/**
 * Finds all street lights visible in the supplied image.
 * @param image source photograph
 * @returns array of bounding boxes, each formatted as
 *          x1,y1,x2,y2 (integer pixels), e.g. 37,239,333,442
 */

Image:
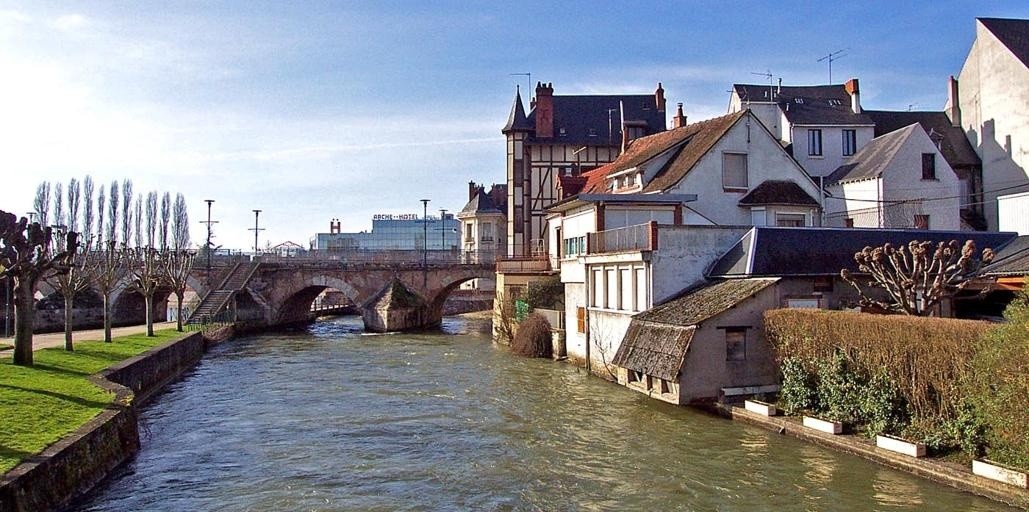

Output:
420,199,431,288
51,224,61,253
204,199,215,286
252,209,262,263
26,212,37,225
4,266,13,337
438,208,449,264
